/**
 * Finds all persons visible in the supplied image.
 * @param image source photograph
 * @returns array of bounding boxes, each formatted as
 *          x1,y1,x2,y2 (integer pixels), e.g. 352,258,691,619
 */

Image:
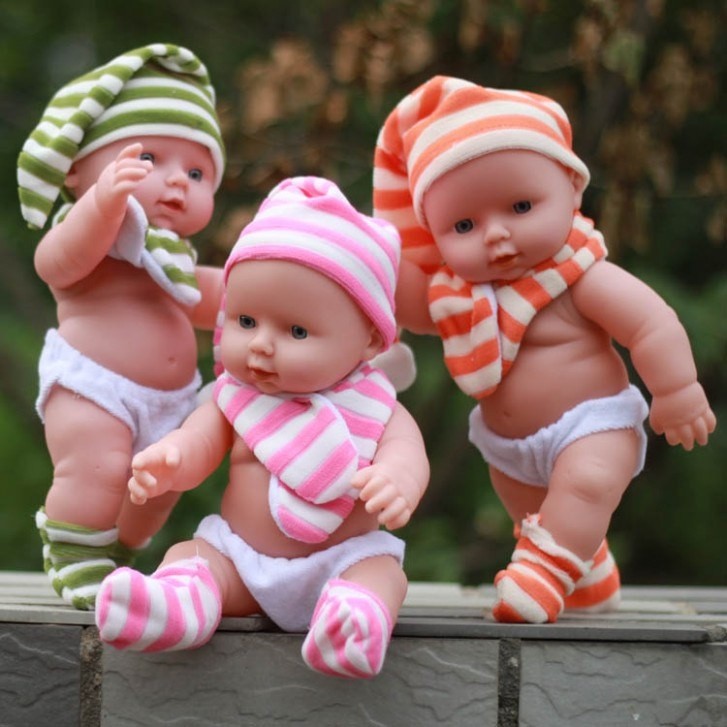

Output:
18,43,232,613
95,176,429,676
372,74,717,621
